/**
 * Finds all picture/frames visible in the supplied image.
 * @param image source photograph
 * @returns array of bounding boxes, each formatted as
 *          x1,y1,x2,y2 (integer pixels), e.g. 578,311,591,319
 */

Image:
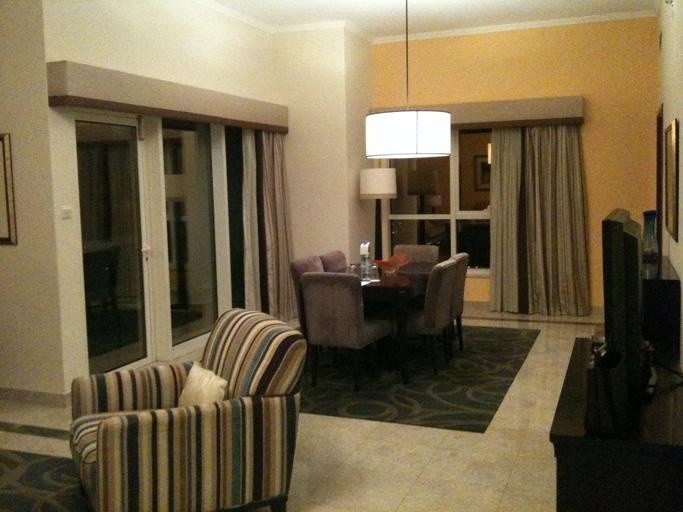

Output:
472,153,492,193
664,117,680,244
0,131,19,248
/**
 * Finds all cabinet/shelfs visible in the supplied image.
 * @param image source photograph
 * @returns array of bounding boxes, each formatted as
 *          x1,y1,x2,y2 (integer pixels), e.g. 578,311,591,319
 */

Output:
549,336,683,511
640,253,681,363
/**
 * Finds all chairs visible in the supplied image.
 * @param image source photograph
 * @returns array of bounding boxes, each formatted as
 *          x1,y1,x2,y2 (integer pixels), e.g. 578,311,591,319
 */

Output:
449,251,470,358
289,255,327,332
392,243,440,271
298,270,391,393
319,249,350,273
405,258,459,377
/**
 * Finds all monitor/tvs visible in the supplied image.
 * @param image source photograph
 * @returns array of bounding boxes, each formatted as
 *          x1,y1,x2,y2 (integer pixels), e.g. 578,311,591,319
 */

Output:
600,206,645,440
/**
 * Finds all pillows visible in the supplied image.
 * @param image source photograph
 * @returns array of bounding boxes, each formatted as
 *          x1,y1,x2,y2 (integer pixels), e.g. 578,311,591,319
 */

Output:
176,357,229,409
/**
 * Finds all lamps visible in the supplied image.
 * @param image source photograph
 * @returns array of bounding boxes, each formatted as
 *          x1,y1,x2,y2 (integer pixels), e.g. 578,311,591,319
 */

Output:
363,0,453,160
359,166,399,261
405,167,437,245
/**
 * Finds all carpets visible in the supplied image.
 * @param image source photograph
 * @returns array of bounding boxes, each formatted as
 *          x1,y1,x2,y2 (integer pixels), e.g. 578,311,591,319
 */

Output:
0,446,92,511
296,323,541,435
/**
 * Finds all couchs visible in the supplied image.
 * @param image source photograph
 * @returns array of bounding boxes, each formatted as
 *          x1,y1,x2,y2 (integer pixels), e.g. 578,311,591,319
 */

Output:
68,306,311,512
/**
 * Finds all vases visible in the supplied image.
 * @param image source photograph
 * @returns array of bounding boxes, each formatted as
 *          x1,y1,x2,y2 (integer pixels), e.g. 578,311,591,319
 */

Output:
640,209,661,266
